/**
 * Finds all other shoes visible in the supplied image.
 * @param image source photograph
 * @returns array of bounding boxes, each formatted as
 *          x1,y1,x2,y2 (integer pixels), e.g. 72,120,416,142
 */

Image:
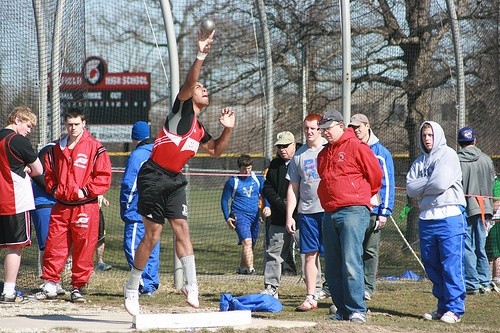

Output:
348,312,366,324
236,267,255,275
0,293,24,304
423,311,442,320
56,284,66,295
440,311,461,323
35,290,57,300
96,262,112,271
490,282,500,293
480,288,490,295
181,286,199,309
466,289,479,295
122,285,139,316
325,313,345,322
258,287,279,300
296,294,317,312
317,291,332,300
365,291,371,301
69,290,86,303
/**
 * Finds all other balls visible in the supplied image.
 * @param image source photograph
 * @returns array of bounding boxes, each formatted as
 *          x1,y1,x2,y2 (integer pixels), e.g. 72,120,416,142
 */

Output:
201,19,215,33
423,313,432,321
329,305,338,316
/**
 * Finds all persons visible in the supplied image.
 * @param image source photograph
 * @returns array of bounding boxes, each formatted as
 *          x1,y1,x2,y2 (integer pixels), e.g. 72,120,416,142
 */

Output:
457,126,500,294
31,108,113,303
260,130,321,301
122,30,235,316
348,114,395,300
119,119,160,296
317,109,384,323
285,113,329,311
221,154,260,275
406,121,467,323
0,105,43,305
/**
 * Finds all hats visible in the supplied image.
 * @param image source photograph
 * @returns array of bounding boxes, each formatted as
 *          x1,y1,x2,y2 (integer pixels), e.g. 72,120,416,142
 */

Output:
347,114,369,128
316,110,343,131
274,131,294,145
131,121,150,141
458,126,476,141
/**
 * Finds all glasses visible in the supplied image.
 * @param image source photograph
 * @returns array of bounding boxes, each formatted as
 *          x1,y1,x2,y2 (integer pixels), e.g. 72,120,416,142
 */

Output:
276,142,293,149
320,123,339,133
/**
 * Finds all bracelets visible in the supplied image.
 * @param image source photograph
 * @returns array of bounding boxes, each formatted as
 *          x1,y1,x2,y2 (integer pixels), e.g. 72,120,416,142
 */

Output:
196,51,209,61
